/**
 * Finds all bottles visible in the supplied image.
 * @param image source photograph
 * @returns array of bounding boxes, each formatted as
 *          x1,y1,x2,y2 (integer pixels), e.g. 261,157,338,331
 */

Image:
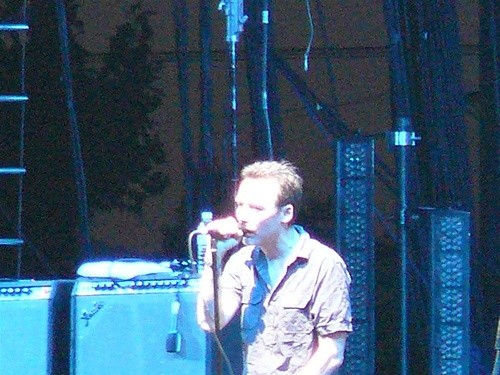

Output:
197,212,212,273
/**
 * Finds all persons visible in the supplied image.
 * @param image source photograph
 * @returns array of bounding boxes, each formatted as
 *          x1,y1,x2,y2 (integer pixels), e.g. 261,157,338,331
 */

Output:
198,160,353,375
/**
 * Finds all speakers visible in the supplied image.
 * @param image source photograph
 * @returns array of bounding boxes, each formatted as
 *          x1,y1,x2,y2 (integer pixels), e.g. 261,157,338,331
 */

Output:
0,274,212,375
